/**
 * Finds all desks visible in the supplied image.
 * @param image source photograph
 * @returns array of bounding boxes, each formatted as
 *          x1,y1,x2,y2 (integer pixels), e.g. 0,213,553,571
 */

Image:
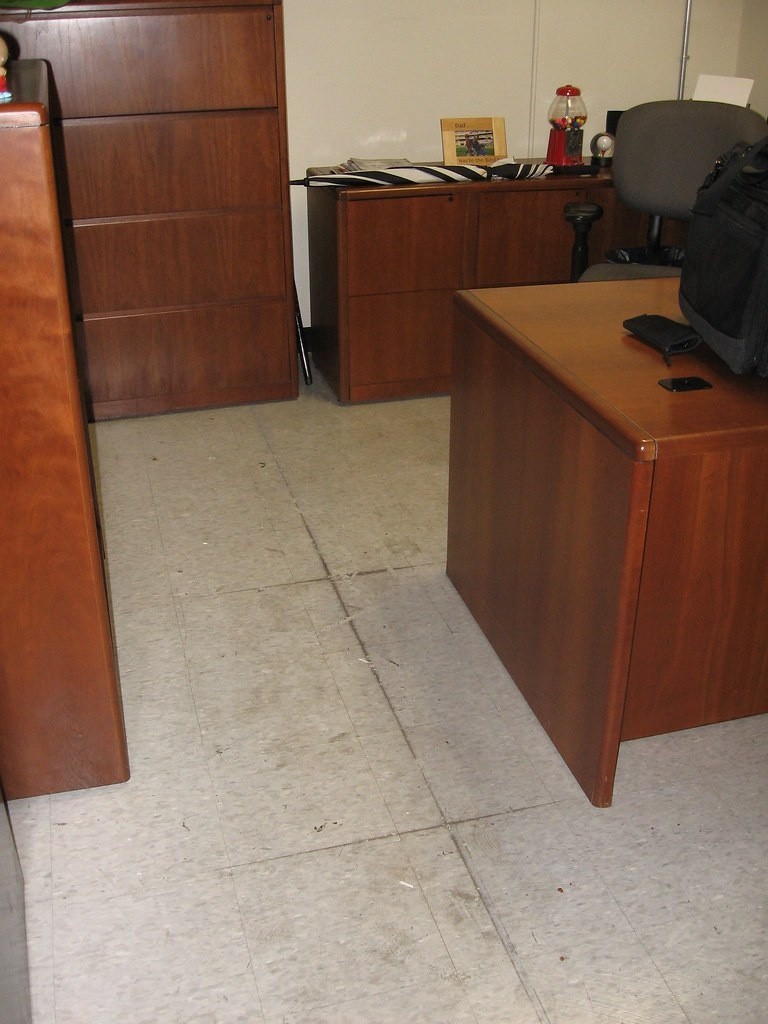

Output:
446,278,767,807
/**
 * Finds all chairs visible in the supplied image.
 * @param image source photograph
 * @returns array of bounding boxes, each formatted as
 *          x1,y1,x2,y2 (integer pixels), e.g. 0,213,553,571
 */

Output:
560,100,767,282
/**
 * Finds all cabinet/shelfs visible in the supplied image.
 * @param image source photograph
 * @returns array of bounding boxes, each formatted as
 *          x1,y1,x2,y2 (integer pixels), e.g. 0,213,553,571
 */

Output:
306,158,654,408
0,0,301,424
0,56,129,807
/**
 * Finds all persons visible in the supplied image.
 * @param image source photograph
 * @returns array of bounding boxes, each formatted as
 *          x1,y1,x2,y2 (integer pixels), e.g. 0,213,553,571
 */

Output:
464,134,484,156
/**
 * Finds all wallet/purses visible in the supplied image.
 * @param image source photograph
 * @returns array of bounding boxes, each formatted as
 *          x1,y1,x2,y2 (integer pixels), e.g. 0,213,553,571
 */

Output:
624,313,702,367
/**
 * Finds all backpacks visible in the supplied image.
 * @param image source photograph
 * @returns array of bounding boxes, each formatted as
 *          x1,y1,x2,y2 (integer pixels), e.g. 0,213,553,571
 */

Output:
678,136,768,378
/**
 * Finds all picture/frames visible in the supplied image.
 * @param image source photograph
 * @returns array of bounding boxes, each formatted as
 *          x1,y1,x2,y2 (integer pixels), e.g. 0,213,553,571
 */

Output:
440,117,508,166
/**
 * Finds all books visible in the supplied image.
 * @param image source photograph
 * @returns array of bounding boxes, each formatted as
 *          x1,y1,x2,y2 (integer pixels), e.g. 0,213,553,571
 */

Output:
331,158,413,174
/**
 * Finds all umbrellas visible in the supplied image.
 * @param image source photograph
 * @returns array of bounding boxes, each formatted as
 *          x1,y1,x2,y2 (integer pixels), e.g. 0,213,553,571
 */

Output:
287,163,599,188
